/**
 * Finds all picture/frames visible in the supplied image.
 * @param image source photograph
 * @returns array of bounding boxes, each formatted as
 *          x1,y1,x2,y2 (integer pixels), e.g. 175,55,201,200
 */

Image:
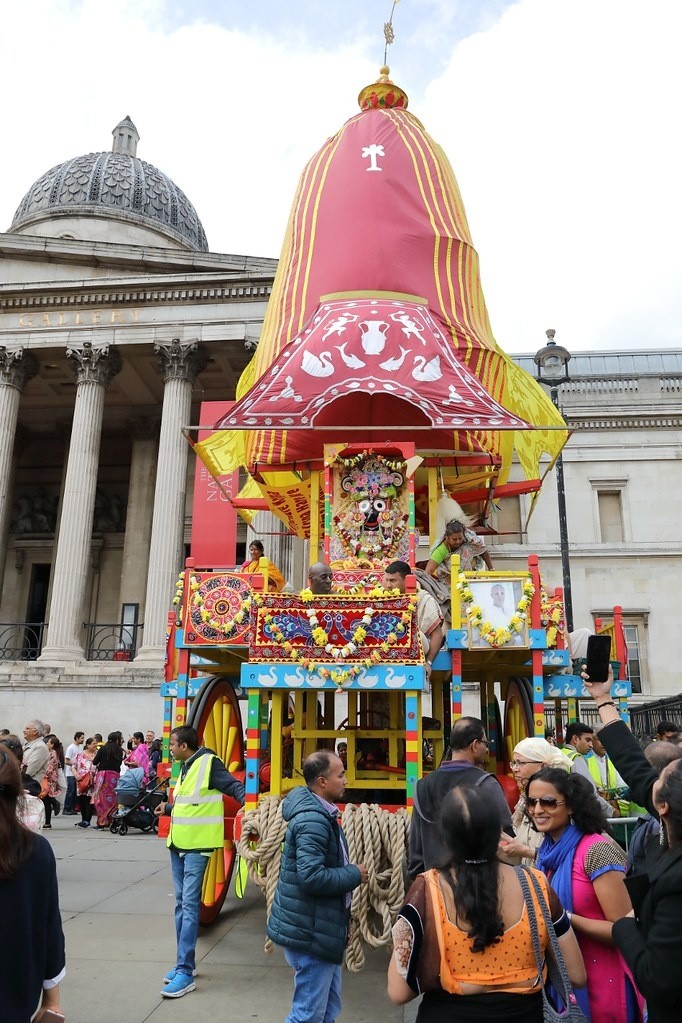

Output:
463,577,532,651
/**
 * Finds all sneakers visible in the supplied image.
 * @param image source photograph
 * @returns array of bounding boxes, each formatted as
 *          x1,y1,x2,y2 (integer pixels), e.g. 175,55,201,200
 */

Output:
160,966,197,997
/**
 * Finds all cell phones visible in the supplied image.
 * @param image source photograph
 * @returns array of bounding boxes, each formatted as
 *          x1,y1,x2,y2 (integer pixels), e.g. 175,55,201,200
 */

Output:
585,634,611,683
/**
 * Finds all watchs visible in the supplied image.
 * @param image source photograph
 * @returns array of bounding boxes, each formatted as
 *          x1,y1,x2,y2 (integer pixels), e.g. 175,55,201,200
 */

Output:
426,658,433,666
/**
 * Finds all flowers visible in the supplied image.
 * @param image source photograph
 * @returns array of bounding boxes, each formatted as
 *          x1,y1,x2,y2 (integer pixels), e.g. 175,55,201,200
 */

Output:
456,569,536,648
539,574,562,648
253,574,419,692
172,571,253,638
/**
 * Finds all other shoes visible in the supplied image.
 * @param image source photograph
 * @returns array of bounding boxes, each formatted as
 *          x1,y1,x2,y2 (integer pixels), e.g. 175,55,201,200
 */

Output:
53,801,60,816
93,825,110,830
39,824,52,829
74,821,91,828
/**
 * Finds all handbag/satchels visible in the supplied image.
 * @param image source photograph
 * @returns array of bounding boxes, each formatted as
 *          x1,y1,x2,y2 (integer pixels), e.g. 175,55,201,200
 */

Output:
514,864,586,1023
38,778,49,798
77,771,94,793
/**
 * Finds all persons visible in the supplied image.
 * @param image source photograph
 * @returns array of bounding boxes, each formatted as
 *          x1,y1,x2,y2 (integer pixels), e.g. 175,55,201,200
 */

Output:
337,742,387,771
425,519,496,629
0,743,66,1023
0,720,163,830
385,561,444,679
386,659,682,1023
154,726,245,998
267,751,369,1023
240,540,285,594
266,689,325,778
484,584,514,631
306,562,340,594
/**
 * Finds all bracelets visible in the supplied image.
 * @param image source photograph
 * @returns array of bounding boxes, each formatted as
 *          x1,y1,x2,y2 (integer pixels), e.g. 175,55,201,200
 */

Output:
291,723,295,729
597,702,616,709
489,568,495,571
566,910,572,924
529,846,535,863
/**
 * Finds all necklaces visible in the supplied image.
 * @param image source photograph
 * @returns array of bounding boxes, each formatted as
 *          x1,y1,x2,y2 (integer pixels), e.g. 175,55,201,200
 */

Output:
249,561,259,583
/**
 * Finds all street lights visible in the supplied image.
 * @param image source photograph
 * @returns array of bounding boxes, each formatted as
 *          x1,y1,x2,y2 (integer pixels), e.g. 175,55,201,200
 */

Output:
533,329,579,633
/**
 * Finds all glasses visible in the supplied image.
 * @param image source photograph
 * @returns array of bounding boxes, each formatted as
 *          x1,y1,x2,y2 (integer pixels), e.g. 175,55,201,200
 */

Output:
478,740,491,747
509,760,543,770
524,797,565,812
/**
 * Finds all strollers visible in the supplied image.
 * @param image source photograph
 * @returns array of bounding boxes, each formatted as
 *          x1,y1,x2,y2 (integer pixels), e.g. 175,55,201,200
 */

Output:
108,766,171,835
